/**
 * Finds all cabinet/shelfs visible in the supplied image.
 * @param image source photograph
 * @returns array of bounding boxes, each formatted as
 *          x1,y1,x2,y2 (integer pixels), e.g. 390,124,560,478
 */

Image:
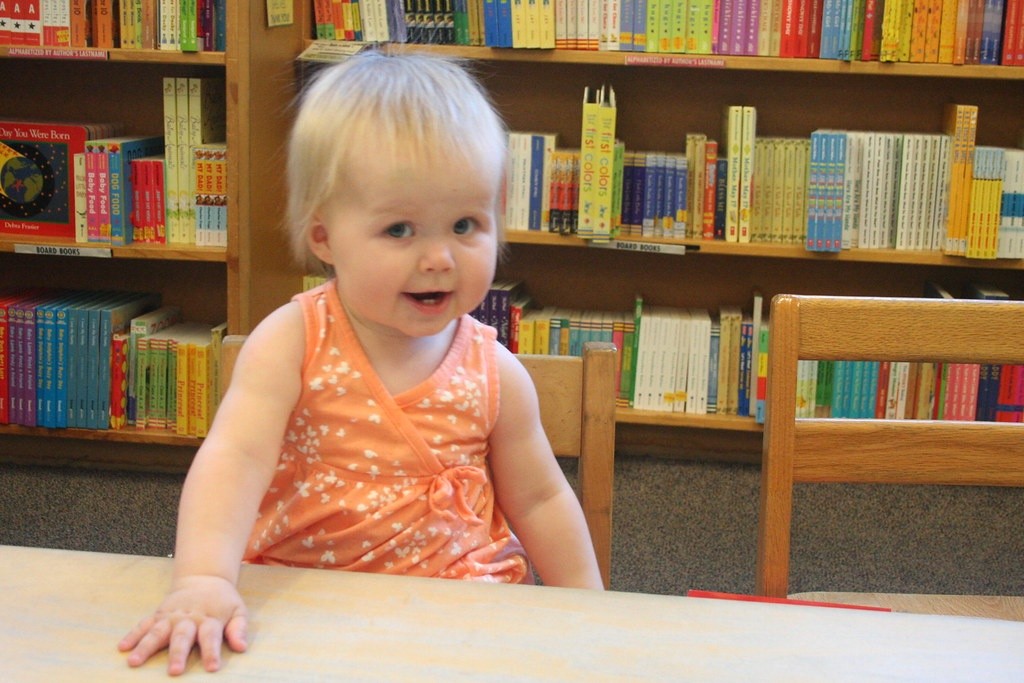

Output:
0,0,1024,486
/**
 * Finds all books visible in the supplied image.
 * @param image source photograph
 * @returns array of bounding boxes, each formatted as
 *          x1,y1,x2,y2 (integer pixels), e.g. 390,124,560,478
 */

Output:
1,0,229,435
300,1,1024,421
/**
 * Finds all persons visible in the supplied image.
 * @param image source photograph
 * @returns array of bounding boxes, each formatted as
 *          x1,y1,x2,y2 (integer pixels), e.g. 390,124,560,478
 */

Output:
118,54,604,676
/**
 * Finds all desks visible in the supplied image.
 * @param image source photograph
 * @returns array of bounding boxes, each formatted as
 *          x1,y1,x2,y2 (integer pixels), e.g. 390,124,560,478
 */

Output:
0,544,1024,683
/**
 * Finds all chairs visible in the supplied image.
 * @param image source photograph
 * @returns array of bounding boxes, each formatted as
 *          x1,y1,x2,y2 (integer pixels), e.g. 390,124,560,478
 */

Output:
754,294,1024,620
